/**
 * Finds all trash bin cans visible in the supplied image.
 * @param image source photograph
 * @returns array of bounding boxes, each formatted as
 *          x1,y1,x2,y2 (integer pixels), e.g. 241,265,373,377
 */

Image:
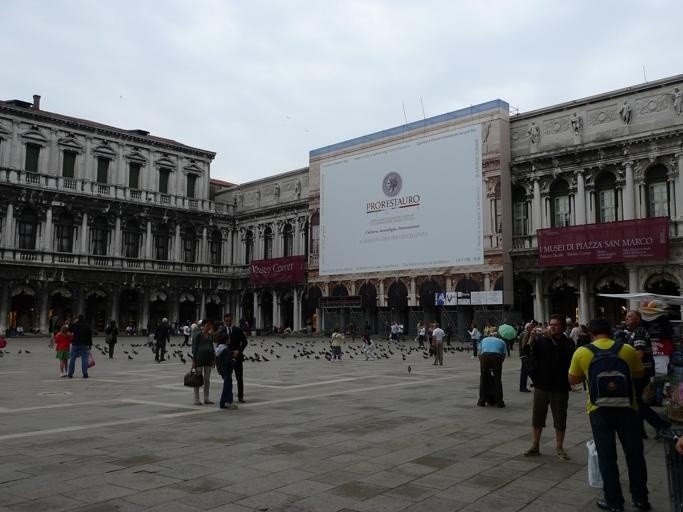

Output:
658,428,683,512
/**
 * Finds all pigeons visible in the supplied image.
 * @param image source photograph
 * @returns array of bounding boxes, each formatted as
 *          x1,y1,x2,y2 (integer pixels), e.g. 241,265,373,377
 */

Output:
407,364,412,374
94,341,192,365
17,348,22,354
25,349,30,354
242,333,474,363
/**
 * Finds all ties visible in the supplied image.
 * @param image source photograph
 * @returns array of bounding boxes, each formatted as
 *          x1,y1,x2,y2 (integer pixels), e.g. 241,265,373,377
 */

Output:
228,327,232,337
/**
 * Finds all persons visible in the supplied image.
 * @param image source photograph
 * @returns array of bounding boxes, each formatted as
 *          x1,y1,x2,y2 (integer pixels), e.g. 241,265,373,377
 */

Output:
48,313,93,379
467,315,591,407
527,121,540,147
672,87,682,117
125,323,136,336
307,318,312,337
617,100,632,126
566,318,654,512
105,320,119,358
173,314,249,408
16,324,24,336
569,112,584,136
435,292,444,304
524,315,575,460
614,311,674,442
414,319,452,366
271,325,277,336
332,320,405,362
385,175,398,194
147,317,170,362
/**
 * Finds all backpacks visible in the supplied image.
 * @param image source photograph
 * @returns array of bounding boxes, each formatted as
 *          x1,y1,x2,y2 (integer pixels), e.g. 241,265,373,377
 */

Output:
581,338,636,412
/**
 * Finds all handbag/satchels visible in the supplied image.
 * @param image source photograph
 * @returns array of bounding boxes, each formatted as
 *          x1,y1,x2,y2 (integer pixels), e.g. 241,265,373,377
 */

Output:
183,367,204,388
87,350,96,369
429,345,436,354
106,334,112,343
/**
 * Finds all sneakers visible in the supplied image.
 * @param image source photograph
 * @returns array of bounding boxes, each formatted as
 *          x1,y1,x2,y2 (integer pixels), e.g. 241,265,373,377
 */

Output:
522,448,541,457
555,452,570,461
60,373,89,379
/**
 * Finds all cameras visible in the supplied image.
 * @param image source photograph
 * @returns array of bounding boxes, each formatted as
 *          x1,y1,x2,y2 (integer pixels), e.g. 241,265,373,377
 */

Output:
534,327,543,334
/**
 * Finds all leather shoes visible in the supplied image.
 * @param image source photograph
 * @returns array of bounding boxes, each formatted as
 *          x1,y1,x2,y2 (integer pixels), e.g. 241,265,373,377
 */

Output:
195,399,245,409
632,500,652,510
597,499,625,512
477,400,485,406
495,400,505,408
655,421,672,440
519,388,531,392
